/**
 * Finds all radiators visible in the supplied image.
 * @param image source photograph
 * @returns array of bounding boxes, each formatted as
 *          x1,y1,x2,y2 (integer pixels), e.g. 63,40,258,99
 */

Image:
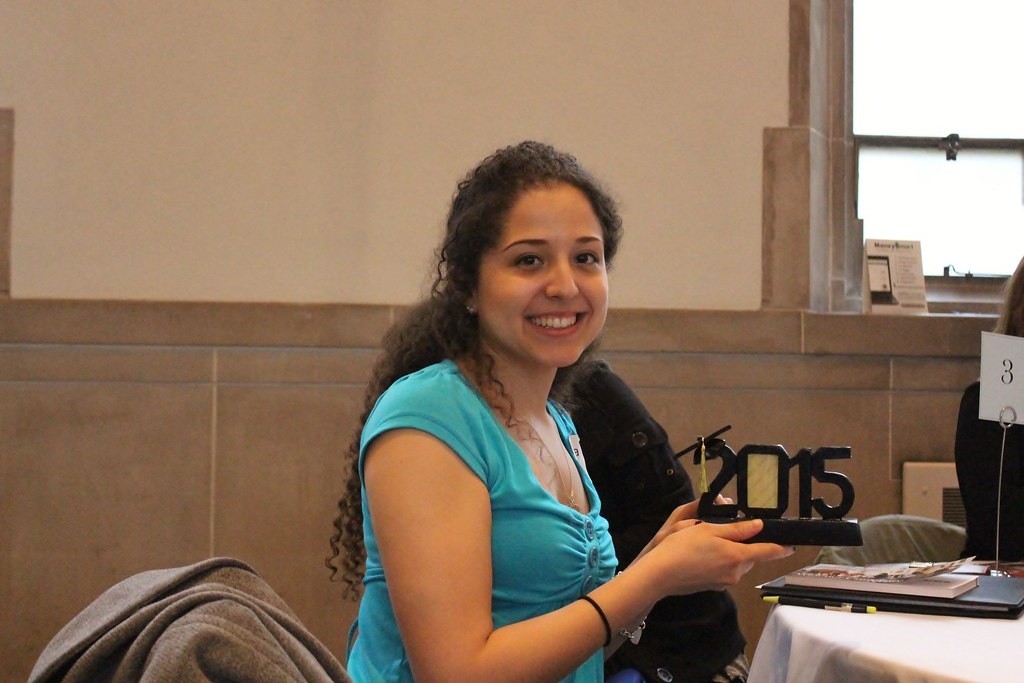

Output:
901,460,968,530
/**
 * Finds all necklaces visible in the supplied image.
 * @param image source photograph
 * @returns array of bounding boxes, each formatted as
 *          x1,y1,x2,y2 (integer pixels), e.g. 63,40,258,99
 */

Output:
464,349,581,514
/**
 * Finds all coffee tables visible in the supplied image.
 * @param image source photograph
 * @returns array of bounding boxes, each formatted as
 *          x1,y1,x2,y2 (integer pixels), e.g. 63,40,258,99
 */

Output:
744,603,1024,683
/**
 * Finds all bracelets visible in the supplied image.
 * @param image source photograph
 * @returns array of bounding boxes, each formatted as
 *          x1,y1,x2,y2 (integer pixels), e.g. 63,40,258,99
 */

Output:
578,596,613,647
612,569,647,645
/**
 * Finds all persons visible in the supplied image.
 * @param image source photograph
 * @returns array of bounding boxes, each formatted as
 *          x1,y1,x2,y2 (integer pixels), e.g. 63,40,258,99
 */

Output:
953,254,1024,563
329,136,800,683
